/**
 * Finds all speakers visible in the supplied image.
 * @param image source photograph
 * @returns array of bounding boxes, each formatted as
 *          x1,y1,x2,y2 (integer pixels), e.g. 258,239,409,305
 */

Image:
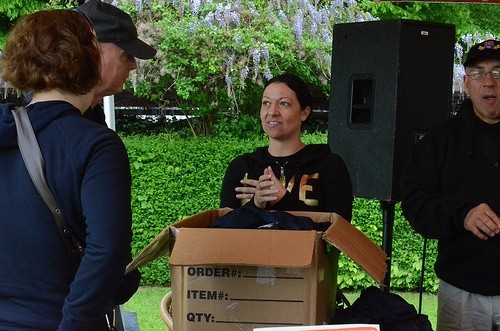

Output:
325,19,456,202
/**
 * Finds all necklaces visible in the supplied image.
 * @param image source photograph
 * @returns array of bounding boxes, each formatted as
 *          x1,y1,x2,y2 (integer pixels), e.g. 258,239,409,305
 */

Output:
268,144,304,175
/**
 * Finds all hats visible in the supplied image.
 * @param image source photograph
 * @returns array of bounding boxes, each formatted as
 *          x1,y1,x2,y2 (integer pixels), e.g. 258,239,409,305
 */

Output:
76,1,157,60
463,39,500,65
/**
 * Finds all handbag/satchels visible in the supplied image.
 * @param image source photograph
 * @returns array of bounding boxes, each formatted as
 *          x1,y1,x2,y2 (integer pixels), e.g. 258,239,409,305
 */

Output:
58,246,123,331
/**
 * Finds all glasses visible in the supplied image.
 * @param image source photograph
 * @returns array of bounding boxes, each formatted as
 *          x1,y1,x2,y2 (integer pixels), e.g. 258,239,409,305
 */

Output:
468,70,500,80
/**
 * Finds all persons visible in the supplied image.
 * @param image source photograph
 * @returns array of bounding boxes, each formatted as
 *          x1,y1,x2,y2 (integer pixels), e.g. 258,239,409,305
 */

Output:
221,71,354,224
0,0,157,331
401,39,500,331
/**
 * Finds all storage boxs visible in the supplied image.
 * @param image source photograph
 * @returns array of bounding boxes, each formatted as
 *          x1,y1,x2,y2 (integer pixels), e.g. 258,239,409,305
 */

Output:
123,207,388,331
